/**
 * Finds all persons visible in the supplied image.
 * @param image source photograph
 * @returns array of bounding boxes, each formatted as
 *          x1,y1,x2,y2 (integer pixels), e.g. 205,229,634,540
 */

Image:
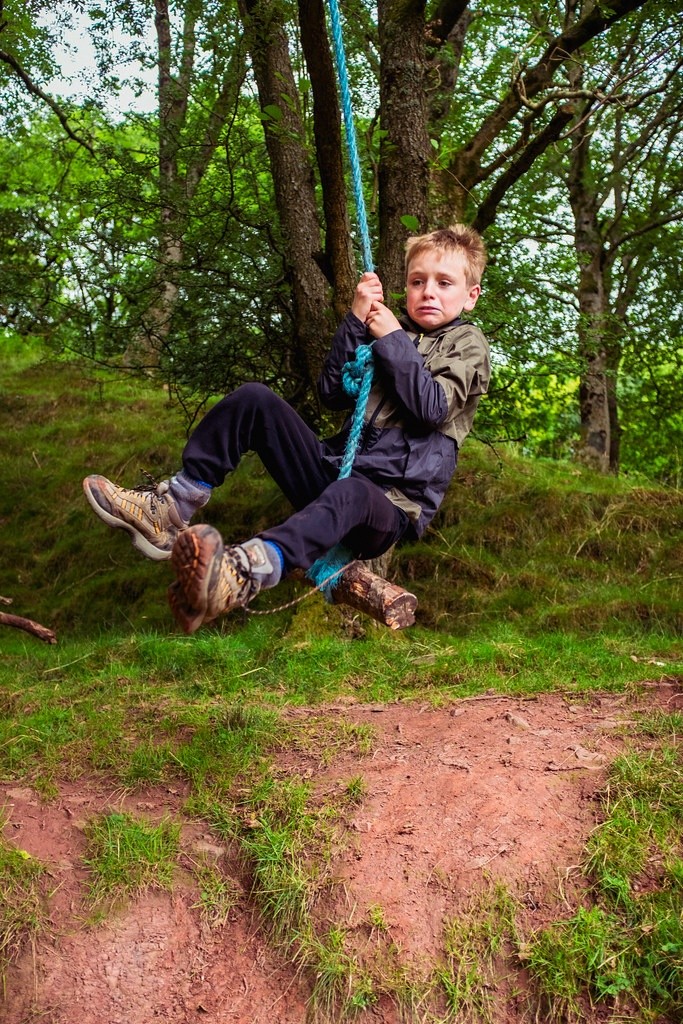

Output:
84,225,491,634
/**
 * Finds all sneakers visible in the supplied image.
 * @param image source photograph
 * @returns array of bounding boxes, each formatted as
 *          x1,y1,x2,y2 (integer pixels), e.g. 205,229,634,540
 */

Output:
83,473,190,560
166,523,273,634
